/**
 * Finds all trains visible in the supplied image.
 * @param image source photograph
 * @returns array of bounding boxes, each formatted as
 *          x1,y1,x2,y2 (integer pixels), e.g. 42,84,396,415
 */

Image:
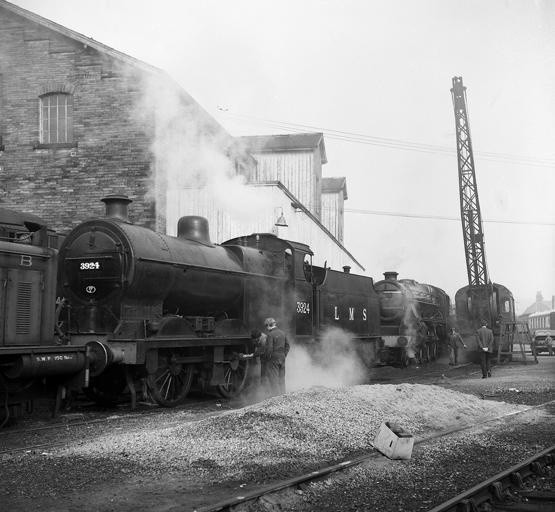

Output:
0,190,452,427
455,283,517,363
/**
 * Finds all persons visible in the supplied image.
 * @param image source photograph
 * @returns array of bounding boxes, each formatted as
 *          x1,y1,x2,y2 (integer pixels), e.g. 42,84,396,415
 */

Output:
545,334,553,355
446,327,467,365
239,329,272,397
474,318,496,378
260,316,291,399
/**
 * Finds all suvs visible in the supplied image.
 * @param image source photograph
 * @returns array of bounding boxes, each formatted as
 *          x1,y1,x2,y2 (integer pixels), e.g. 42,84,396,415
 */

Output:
531,329,555,355
